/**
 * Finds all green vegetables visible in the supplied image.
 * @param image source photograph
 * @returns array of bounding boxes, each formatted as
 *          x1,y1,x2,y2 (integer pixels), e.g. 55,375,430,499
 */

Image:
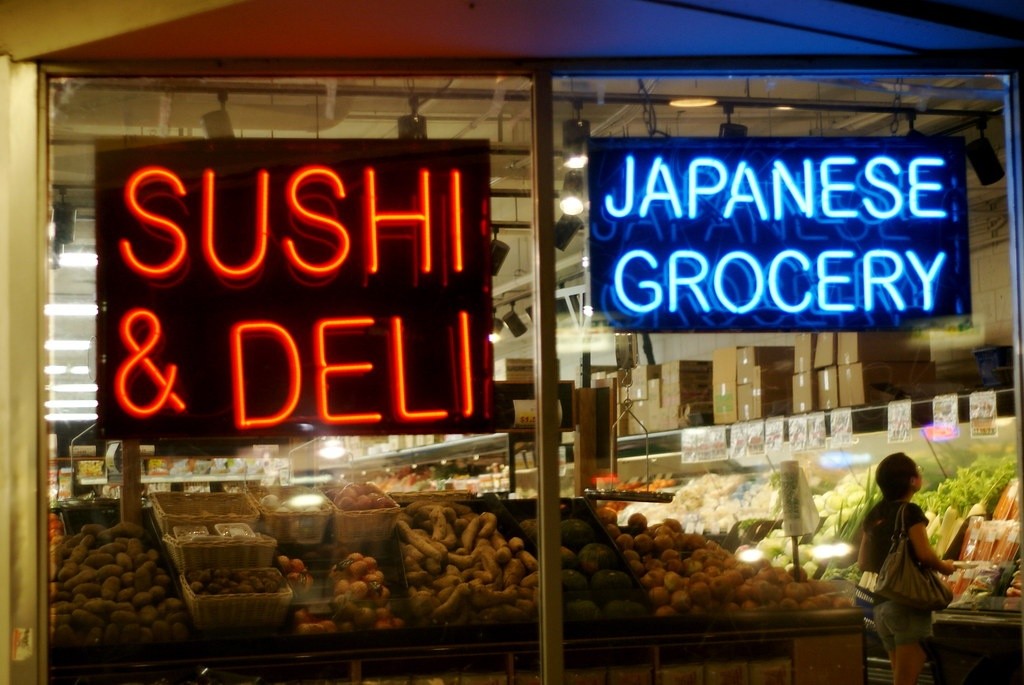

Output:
914,456,1016,561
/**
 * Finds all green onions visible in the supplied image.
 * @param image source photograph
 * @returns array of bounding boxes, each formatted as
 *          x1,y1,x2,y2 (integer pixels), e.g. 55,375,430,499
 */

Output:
821,473,882,581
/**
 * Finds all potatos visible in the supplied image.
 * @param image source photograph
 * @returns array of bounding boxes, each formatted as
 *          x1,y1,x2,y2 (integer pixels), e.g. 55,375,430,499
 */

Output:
399,502,543,631
186,568,285,595
51,521,191,647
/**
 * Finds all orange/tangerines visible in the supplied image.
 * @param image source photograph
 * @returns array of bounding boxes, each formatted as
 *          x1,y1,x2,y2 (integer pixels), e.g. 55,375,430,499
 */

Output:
49,512,63,543
274,551,405,636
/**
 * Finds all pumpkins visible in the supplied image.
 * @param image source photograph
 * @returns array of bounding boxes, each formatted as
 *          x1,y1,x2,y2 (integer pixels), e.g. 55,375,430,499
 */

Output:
519,516,649,620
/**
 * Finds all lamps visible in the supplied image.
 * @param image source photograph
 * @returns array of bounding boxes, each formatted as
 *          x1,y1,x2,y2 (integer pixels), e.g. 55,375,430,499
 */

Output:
504,302,527,338
202,91,233,138
491,226,511,277
561,101,594,171
55,189,77,243
397,97,427,138
965,113,1005,186
491,308,503,341
719,100,748,138
555,214,583,252
560,172,585,215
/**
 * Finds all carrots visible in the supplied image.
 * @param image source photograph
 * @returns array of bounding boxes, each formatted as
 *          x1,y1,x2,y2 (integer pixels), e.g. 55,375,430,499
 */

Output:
935,479,1021,611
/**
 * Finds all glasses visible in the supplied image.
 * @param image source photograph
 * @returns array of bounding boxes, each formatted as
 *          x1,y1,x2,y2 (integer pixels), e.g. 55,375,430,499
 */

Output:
916,464,924,474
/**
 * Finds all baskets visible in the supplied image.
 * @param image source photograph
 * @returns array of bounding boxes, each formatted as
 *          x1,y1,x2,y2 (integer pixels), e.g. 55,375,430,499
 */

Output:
150,481,401,631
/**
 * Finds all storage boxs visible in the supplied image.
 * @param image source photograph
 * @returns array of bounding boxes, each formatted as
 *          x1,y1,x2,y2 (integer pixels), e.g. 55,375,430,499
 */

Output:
388,327,1013,492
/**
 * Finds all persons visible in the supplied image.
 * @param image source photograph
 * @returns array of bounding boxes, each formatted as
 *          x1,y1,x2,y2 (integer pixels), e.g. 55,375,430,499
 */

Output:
857,453,957,685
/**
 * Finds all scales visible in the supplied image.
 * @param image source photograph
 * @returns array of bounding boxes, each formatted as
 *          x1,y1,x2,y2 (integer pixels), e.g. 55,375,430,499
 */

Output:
584,364,676,504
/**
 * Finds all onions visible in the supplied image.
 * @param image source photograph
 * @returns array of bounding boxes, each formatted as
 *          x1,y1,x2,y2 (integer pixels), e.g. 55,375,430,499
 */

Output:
594,508,845,618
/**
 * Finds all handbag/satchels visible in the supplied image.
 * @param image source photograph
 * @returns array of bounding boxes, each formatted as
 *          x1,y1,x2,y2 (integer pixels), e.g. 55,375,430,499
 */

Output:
874,503,954,610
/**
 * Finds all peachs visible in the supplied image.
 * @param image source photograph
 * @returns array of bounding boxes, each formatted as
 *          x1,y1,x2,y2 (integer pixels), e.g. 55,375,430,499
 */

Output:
326,483,389,511
